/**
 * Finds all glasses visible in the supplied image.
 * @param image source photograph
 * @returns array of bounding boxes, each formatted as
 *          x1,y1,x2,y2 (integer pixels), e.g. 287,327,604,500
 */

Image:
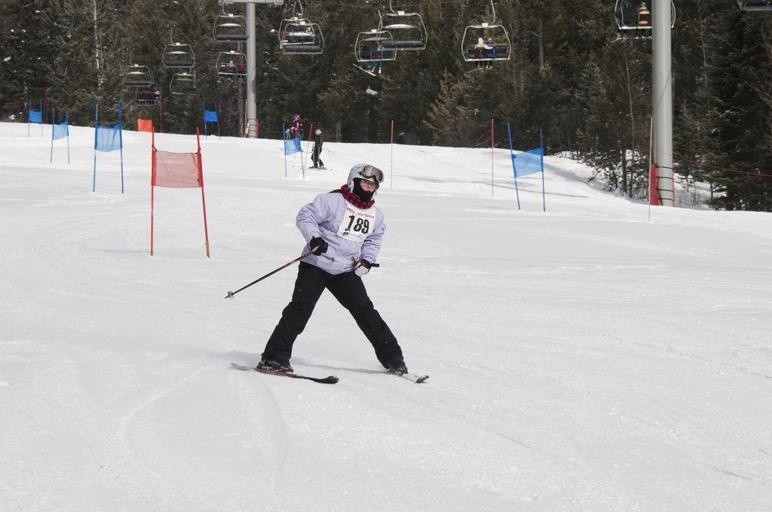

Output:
359,165,383,183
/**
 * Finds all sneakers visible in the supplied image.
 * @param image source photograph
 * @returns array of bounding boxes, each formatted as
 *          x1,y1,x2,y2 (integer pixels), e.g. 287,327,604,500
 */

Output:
257,359,293,373
389,362,408,376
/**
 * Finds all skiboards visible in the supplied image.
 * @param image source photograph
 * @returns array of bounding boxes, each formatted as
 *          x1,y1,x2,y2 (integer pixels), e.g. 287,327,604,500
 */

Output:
231,363,429,384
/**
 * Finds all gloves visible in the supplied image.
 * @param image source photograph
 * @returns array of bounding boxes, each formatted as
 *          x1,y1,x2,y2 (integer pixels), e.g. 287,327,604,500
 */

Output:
352,259,371,277
310,237,328,256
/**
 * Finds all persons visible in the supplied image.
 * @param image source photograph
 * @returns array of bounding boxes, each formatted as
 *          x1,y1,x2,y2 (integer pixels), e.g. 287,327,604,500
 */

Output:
239,60,246,72
310,130,324,169
474,37,496,72
291,114,302,153
306,27,313,42
616,2,650,41
258,163,407,376
372,45,385,74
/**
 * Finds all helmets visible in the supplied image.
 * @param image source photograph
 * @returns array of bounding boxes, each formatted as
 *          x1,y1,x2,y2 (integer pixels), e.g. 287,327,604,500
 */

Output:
347,163,383,194
315,129,321,135
